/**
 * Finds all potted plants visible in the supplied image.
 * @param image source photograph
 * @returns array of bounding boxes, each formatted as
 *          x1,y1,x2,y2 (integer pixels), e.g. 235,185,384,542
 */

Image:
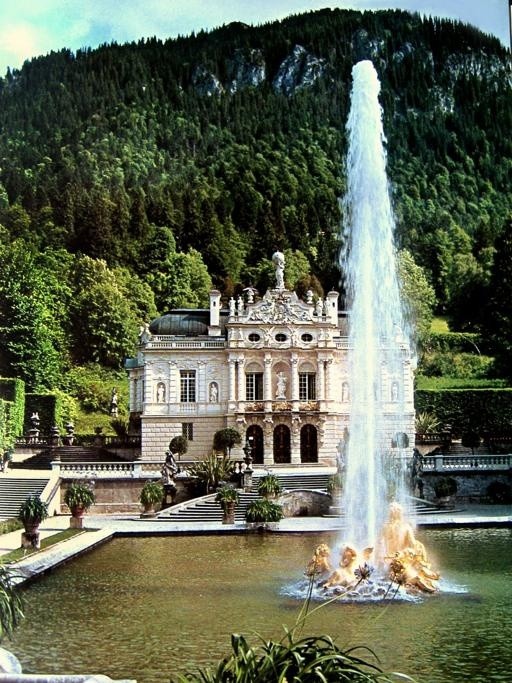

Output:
18,427,282,553
429,474,458,504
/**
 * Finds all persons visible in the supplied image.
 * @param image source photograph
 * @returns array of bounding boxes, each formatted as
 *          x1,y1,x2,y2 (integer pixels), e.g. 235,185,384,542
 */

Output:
209,383,217,401
412,448,424,478
391,383,398,401
275,260,287,289
228,296,236,316
274,370,288,398
157,384,165,403
342,383,350,402
315,296,324,317
324,296,331,315
237,295,244,315
161,450,179,485
